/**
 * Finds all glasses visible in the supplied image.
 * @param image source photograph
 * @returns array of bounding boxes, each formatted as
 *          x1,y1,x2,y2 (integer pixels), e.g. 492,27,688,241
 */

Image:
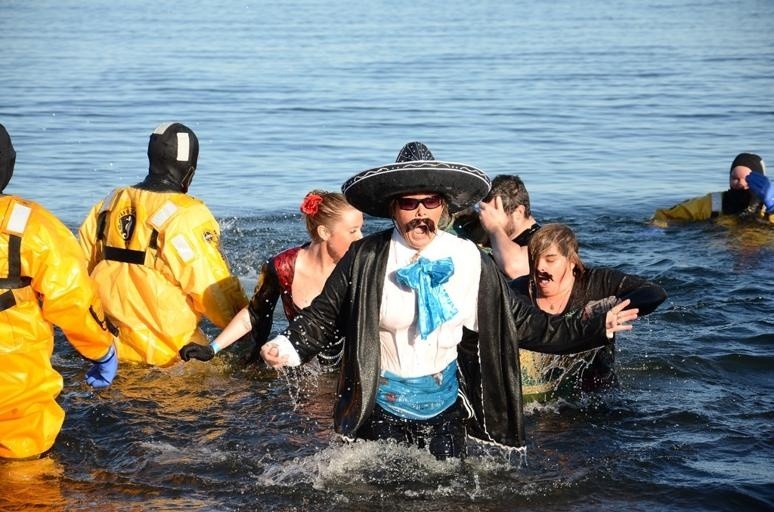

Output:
395,194,443,210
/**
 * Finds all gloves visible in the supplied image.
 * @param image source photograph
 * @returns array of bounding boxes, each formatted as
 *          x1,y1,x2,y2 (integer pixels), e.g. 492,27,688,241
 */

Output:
84,345,118,388
745,170,774,215
179,342,214,362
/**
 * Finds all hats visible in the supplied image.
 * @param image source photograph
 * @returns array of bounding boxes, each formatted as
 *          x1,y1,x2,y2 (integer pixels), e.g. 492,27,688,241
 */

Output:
341,141,492,219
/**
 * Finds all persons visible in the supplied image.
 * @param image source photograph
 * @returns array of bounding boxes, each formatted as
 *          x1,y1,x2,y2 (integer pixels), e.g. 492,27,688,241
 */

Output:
78,123,250,370
0,125,116,512
650,153,774,245
183,189,365,373
440,176,667,411
261,142,639,456
